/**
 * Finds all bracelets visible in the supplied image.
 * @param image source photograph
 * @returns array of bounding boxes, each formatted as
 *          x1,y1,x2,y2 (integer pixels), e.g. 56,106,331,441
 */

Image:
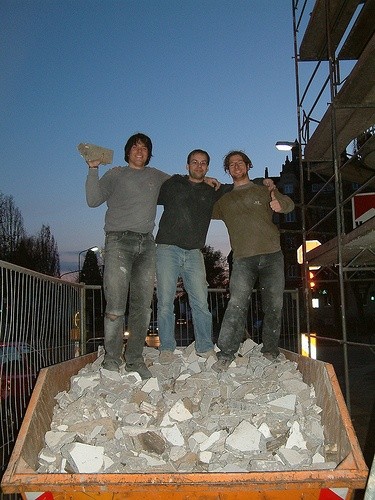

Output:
89,165,99,169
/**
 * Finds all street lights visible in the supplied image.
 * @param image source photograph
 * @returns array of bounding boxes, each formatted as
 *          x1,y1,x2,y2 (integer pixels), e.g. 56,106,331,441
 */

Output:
274,139,353,157
78,246,99,283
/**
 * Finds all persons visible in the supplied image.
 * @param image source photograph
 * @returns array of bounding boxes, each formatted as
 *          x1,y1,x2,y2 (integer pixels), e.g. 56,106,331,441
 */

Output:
111,148,275,365
211,144,295,374
84,134,222,382
